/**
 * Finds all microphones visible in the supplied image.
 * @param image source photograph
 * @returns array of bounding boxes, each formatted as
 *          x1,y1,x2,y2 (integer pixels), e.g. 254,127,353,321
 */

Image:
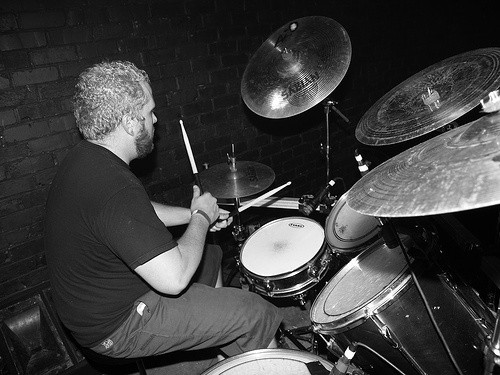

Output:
300,179,334,217
355,153,400,249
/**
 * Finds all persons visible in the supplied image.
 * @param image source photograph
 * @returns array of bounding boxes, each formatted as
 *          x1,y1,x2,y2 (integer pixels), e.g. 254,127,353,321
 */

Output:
43,60,286,361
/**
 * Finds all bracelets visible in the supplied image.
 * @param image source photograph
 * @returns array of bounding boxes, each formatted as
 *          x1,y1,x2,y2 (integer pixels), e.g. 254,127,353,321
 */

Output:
191,209,211,225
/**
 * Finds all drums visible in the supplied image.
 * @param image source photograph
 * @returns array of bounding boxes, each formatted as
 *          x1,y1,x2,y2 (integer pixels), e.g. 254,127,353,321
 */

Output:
199,349,334,375
323,192,401,266
238,216,332,298
309,232,496,375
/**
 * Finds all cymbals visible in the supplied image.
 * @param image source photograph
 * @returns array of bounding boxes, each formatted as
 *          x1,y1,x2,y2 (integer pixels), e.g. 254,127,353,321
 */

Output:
194,161,276,199
240,15,352,120
345,112,500,217
355,47,500,147
